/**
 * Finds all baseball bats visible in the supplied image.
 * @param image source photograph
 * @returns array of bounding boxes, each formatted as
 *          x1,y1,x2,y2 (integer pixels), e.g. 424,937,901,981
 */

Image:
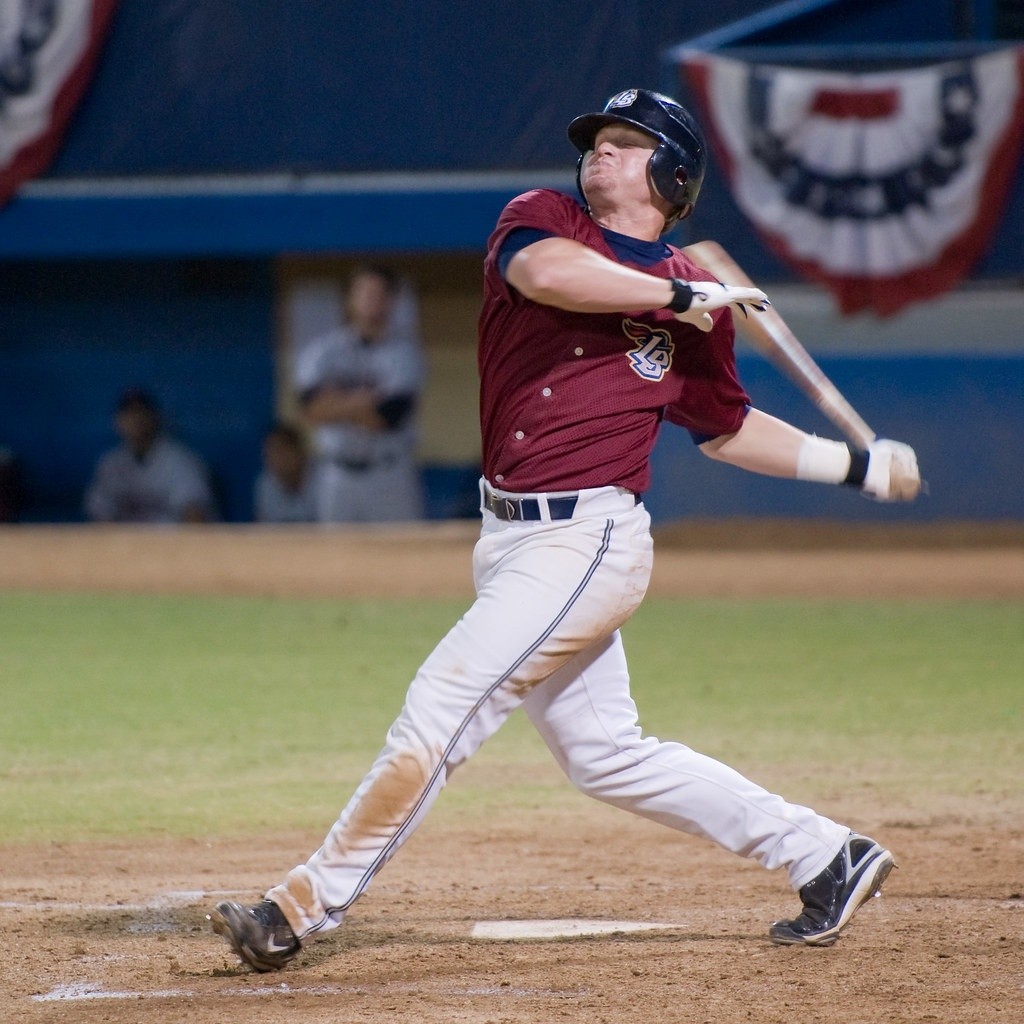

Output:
685,236,922,501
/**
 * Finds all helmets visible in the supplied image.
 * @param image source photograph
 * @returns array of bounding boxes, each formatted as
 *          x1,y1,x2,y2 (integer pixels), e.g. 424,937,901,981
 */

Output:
566,88,709,219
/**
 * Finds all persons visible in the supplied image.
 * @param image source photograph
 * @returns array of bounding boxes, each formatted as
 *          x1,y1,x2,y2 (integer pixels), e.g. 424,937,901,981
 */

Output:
211,85,922,975
0,380,210,527
253,258,429,523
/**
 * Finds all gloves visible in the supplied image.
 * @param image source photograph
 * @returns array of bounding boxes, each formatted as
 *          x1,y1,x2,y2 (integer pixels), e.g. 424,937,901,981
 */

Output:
837,438,920,503
659,277,772,332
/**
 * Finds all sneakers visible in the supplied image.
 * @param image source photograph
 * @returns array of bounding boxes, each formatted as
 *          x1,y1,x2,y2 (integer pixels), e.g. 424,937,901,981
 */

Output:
210,897,300,972
768,829,898,946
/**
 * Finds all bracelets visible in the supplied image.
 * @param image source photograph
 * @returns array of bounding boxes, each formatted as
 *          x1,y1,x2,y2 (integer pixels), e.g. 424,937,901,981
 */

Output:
659,275,693,313
838,441,870,488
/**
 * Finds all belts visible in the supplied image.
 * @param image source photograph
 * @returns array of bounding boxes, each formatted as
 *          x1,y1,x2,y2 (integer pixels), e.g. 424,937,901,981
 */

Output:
322,453,397,472
483,481,644,520
